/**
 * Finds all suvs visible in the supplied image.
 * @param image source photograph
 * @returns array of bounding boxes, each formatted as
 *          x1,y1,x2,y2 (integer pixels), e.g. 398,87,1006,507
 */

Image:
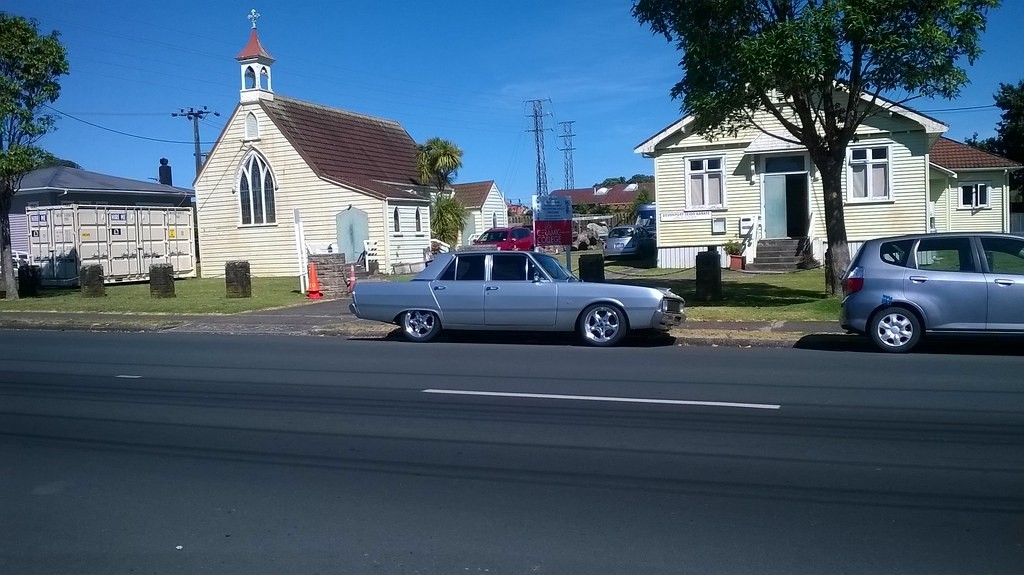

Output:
472,226,534,254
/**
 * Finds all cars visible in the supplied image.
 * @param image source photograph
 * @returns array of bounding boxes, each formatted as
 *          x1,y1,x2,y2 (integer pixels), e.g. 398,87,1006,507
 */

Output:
840,232,1024,352
349,250,687,347
604,225,656,260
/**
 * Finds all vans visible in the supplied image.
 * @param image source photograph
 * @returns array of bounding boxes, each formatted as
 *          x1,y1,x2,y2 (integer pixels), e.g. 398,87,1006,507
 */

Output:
635,202,656,235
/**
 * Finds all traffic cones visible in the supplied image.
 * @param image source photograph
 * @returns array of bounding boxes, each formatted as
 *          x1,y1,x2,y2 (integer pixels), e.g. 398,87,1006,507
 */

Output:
305,261,324,291
304,291,323,299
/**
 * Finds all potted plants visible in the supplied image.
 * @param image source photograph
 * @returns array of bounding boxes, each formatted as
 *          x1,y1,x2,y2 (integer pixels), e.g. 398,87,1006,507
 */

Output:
723,233,746,271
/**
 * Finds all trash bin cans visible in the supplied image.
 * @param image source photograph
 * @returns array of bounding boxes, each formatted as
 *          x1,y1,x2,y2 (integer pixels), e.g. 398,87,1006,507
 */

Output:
80,264,105,297
149,263,176,297
18,266,40,292
578,253,607,283
225,260,252,298
696,251,722,292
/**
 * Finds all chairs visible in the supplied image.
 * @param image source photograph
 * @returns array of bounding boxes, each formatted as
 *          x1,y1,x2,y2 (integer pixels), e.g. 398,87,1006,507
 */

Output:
493,232,514,242
959,244,994,273
